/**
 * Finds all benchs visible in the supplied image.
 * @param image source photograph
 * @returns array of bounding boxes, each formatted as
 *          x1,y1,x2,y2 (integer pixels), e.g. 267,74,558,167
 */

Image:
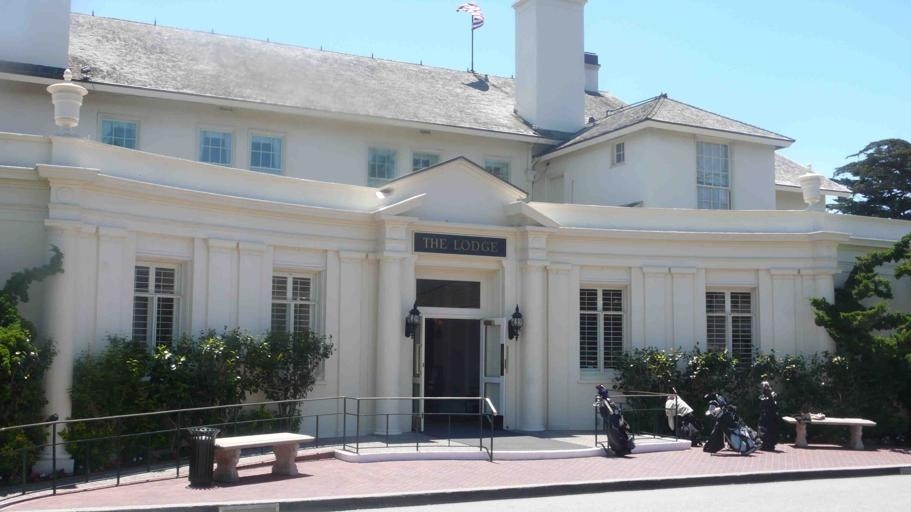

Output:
213,430,316,483
781,415,878,450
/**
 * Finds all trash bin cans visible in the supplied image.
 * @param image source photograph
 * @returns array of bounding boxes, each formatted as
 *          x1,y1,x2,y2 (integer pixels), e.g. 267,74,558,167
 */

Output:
187,426,220,486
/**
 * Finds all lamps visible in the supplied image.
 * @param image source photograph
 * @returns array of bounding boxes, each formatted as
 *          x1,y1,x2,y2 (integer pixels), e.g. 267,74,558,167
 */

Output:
404,299,421,341
508,304,524,342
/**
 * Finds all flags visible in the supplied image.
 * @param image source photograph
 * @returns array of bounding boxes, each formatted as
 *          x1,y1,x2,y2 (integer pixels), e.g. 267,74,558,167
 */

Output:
456,1,483,29
